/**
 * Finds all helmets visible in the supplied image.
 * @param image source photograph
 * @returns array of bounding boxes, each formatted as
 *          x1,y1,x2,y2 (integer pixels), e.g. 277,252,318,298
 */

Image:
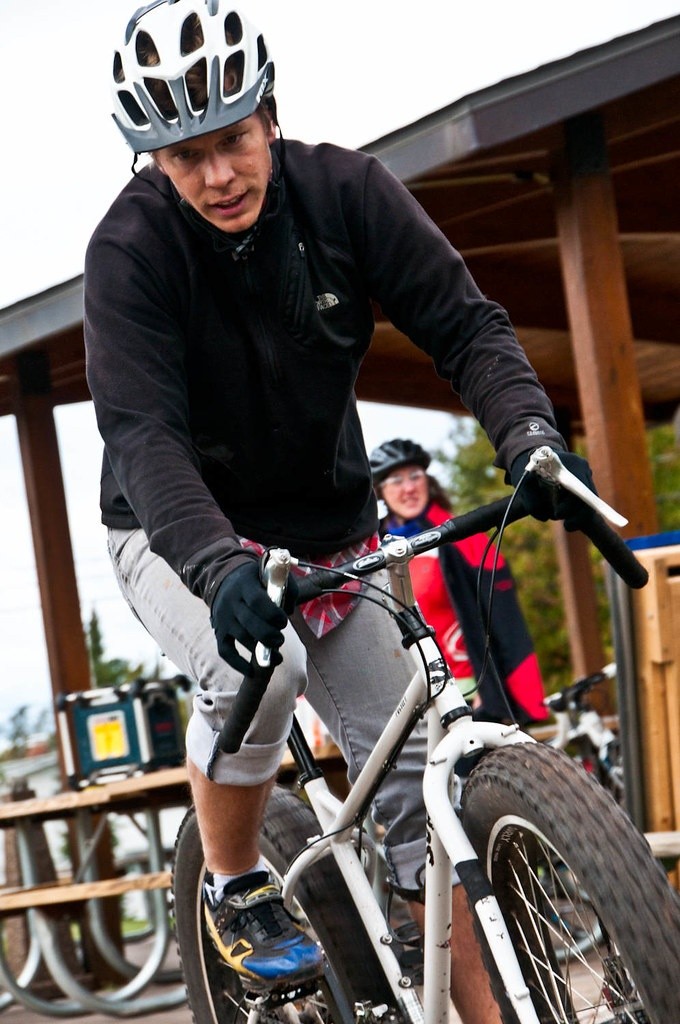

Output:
368,440,431,483
106,0,276,155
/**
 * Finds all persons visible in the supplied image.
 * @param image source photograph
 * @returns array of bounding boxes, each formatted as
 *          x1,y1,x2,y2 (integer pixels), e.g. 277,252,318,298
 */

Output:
76,0,604,1024
365,436,553,735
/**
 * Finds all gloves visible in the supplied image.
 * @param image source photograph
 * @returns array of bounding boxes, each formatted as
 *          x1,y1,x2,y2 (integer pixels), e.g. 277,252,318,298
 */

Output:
209,564,301,671
506,450,601,533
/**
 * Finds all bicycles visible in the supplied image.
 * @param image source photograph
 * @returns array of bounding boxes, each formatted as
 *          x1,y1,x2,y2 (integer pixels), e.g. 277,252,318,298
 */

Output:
149,450,679,1024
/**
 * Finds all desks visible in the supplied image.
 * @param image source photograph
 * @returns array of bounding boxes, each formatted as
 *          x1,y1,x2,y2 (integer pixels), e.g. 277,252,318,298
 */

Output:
0,765,219,1018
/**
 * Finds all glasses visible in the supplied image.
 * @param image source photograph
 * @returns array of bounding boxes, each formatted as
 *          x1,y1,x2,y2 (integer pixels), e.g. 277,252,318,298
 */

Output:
381,471,427,489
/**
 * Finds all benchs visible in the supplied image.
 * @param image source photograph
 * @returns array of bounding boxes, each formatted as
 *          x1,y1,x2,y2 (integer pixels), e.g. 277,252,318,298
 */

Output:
0,875,179,1020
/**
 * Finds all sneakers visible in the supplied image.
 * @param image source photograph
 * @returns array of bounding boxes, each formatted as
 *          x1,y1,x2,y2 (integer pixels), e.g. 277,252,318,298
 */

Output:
195,869,326,994
